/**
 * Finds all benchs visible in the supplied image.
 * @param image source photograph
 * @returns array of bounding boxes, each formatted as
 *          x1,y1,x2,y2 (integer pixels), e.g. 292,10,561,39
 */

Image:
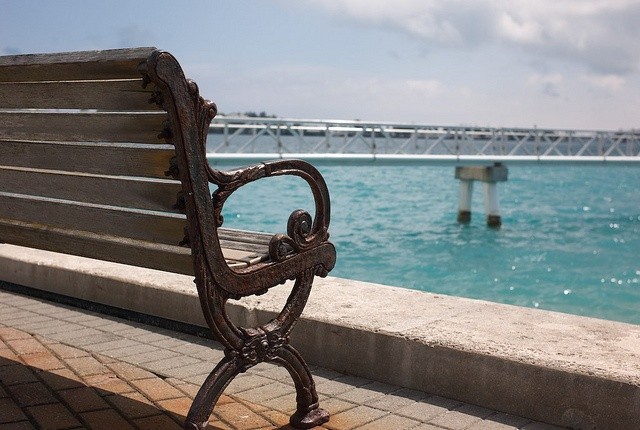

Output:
0,46,337,429
